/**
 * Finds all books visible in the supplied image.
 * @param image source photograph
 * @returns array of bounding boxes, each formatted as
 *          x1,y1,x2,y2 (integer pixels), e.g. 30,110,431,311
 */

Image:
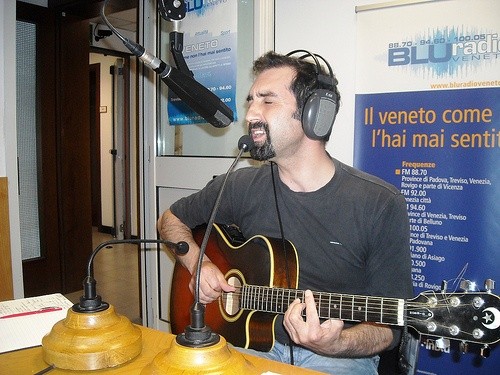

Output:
0,292,73,353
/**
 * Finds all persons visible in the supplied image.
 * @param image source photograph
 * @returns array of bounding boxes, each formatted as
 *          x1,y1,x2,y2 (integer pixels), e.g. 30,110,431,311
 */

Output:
157,51,412,375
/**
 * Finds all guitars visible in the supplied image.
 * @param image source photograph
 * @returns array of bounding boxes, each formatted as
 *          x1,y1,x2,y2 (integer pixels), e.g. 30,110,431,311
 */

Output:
170,222,500,368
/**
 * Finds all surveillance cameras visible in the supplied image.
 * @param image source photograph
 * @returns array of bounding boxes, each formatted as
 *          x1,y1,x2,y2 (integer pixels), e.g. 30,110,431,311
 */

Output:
97,30,113,36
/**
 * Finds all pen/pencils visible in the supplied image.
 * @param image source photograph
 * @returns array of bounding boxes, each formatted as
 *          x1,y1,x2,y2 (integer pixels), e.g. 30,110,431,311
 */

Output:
0,306,62,319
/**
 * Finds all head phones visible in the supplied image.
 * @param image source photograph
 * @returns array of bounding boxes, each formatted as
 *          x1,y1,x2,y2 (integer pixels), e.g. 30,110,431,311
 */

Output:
286,50,338,140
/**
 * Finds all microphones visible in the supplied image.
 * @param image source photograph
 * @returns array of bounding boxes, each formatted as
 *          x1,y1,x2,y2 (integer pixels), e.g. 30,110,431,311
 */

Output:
183,136,255,341
80,239,189,308
125,40,235,128
